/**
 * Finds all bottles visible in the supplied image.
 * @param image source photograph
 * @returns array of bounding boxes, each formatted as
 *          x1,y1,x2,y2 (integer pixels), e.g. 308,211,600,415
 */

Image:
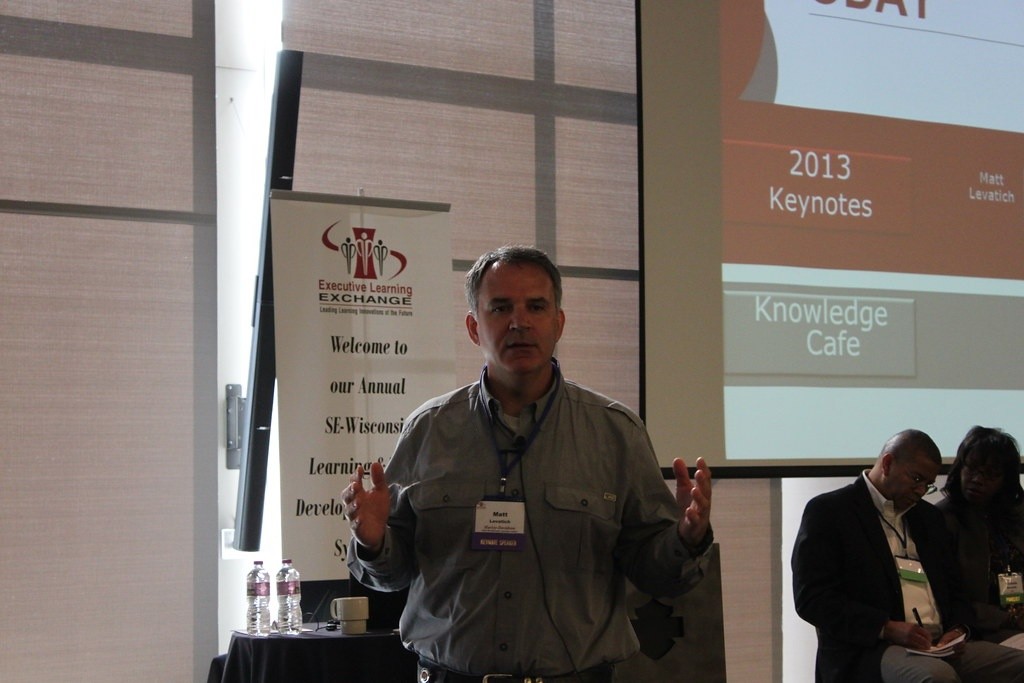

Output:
275,558,302,635
246,560,271,637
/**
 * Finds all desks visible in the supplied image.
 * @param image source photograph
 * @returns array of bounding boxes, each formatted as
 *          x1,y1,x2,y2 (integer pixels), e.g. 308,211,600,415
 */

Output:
206,623,417,683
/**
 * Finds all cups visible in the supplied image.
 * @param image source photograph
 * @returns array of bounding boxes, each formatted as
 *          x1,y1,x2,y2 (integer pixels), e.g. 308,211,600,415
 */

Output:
330,597,370,635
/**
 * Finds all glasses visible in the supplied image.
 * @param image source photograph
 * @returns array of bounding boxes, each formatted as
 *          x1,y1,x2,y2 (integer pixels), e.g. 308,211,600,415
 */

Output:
963,461,1007,483
891,454,937,495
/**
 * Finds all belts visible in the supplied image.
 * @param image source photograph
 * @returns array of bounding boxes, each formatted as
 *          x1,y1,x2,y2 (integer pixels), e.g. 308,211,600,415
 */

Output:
417,658,611,683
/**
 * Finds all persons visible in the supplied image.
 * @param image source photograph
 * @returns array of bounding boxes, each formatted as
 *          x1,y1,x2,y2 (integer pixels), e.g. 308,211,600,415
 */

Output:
933,428,1024,650
791,430,1024,683
341,241,714,683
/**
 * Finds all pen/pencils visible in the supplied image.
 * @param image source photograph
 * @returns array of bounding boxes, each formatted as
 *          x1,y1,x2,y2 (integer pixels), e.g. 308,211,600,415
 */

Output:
912,608,924,628
